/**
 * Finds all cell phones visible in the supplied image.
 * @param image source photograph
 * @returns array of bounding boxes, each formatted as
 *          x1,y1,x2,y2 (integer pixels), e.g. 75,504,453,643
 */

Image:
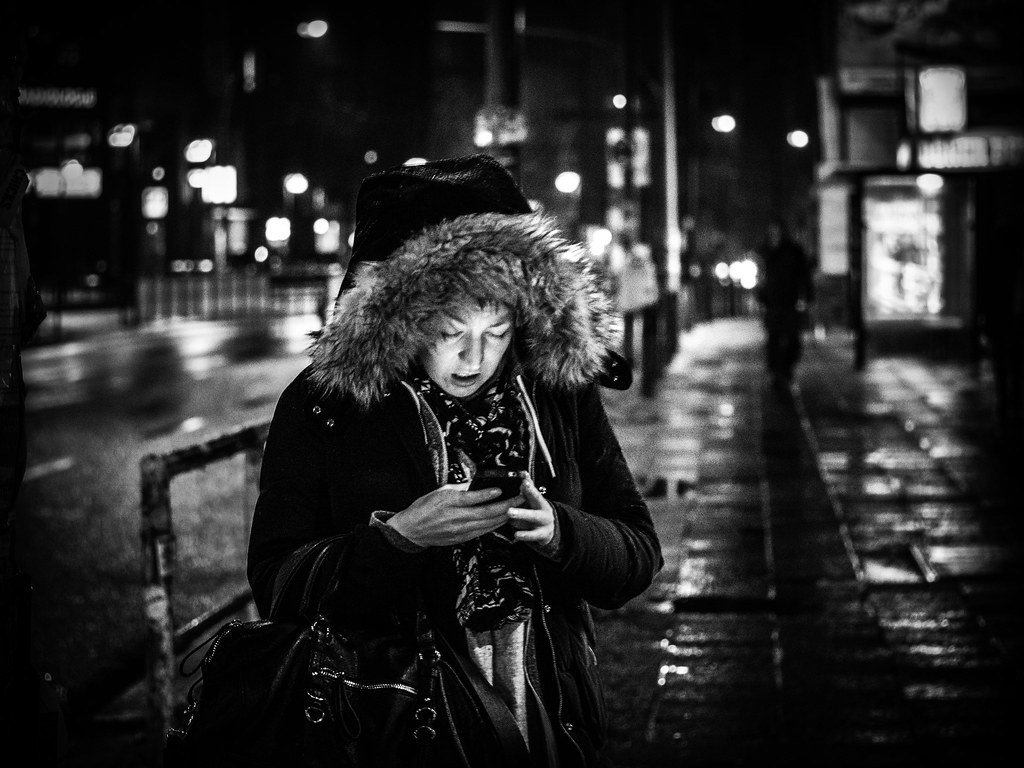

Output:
467,471,524,505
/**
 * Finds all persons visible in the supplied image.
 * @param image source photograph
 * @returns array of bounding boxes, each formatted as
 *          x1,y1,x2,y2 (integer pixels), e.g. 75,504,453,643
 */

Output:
754,216,816,385
246,153,664,768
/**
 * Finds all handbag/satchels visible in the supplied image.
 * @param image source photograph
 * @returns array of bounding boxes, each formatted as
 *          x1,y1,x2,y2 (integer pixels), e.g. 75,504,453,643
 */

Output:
158,531,470,768
